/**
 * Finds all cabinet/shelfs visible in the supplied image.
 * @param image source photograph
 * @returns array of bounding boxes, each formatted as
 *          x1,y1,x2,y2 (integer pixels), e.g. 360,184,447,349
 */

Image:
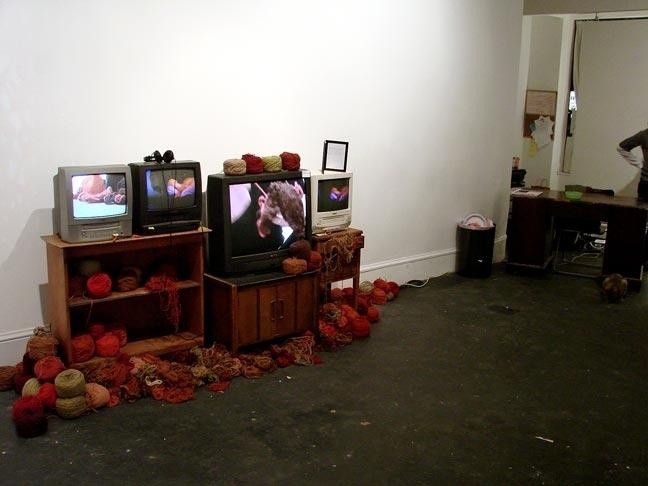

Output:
39,227,213,369
203,266,321,357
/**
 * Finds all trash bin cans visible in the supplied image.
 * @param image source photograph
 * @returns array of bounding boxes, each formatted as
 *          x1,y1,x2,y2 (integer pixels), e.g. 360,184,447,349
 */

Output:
455,222,497,280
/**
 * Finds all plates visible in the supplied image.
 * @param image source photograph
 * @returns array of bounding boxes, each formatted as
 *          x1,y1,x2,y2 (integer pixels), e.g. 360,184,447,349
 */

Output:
464,214,486,227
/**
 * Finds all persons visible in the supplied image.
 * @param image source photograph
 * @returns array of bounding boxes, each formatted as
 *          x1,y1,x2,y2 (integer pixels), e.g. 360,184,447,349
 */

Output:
232,191,285,257
616,124,647,205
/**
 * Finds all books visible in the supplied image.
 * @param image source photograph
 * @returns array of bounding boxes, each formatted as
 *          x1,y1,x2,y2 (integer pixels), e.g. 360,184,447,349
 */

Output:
509,187,544,198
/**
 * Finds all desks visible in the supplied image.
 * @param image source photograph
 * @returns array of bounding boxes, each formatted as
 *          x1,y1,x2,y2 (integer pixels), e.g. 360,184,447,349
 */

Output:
506,187,648,294
311,229,364,347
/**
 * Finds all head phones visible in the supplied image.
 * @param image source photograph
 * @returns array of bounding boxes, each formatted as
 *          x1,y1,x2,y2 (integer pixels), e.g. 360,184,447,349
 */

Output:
144,150,174,164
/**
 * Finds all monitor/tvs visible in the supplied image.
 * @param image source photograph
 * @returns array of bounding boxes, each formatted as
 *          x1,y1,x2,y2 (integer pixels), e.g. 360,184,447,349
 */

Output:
207,169,311,275
129,160,202,236
312,172,353,234
54,164,134,243
